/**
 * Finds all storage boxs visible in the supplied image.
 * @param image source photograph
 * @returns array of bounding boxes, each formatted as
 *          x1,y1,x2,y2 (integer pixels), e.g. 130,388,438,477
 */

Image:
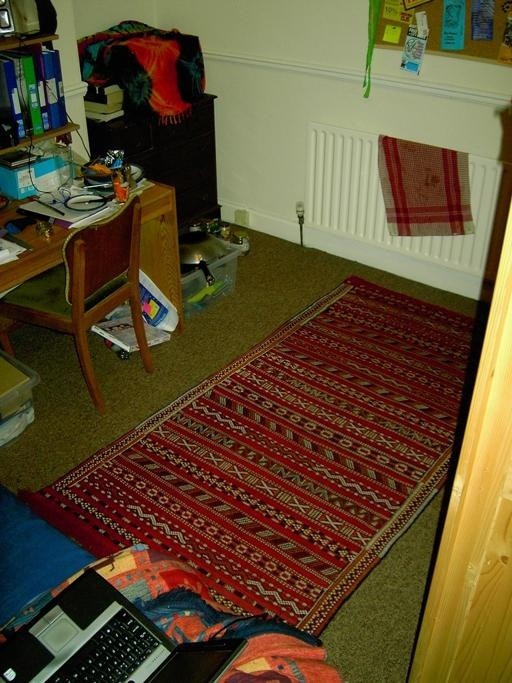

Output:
0,347,41,426
180,243,241,314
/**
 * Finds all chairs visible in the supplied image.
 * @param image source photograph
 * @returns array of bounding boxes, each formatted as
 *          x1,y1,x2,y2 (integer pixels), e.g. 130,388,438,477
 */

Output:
0,194,152,417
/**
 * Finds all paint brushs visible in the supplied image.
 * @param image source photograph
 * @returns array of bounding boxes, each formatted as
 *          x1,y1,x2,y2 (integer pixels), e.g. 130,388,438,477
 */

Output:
88,155,101,167
34,198,65,216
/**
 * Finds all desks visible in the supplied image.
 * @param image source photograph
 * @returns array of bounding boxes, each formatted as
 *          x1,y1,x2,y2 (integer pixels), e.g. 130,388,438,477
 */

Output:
0,174,182,330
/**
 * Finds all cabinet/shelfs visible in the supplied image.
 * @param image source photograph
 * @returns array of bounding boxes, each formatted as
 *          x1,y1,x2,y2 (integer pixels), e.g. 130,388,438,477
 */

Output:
0,34,80,154
85,91,221,236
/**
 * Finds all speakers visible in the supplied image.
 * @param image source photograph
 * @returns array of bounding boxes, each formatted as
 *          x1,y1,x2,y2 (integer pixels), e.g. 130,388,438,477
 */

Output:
0,0,57,37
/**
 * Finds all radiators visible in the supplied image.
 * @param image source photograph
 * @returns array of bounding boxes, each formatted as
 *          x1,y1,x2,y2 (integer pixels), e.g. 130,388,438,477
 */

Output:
304,121,504,275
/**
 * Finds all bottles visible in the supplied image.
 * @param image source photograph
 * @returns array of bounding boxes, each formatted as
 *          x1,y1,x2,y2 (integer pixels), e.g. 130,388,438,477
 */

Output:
219,221,230,238
103,337,130,361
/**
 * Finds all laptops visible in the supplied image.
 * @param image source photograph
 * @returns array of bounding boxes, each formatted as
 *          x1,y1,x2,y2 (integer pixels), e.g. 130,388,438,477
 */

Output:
0,566,250,683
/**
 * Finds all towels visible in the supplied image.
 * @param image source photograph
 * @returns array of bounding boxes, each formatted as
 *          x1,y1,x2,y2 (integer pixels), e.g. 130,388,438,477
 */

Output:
378,133,478,237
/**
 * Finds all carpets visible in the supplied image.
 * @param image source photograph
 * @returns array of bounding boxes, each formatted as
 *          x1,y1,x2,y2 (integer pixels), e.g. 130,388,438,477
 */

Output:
16,273,475,641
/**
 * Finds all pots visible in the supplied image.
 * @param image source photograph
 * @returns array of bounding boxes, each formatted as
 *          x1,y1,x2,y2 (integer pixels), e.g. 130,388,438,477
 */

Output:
178,230,225,285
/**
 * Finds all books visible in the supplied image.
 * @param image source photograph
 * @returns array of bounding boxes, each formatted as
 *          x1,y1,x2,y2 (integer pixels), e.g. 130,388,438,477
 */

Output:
1,44,69,149
1,356,31,399
17,176,156,230
90,316,171,352
83,82,127,122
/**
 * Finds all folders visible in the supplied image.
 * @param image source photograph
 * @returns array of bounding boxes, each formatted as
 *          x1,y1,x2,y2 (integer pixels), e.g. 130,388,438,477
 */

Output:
0,48,66,140
